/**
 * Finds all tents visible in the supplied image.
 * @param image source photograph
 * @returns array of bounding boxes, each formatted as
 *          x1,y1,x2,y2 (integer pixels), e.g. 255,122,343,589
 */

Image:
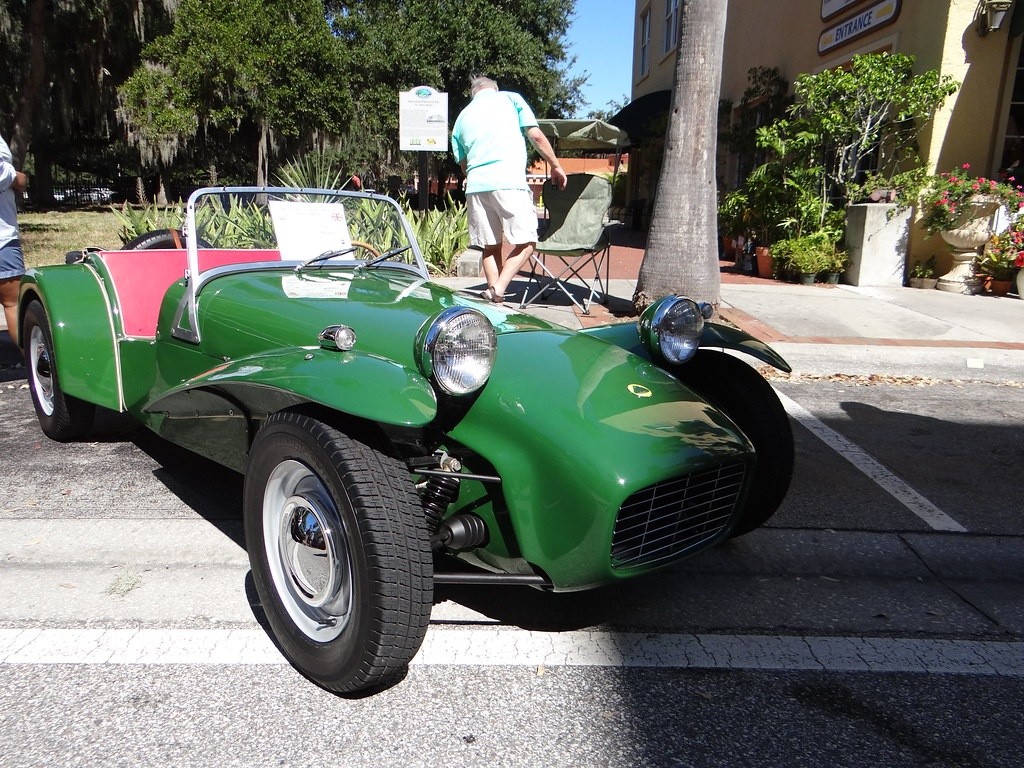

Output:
537,118,631,187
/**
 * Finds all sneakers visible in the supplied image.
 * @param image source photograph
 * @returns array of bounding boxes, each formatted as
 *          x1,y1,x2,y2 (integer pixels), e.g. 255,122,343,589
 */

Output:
480,286,504,306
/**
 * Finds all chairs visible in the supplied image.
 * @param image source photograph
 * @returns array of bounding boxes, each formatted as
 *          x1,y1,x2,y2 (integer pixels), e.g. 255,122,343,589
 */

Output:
518,119,633,316
609,198,655,232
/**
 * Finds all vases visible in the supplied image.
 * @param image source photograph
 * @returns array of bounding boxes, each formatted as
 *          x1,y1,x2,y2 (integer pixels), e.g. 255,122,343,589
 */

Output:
1015,267,1024,300
940,193,1005,293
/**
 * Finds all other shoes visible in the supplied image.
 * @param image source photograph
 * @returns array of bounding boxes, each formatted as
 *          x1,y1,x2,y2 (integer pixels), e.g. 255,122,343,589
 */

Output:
20,358,28,369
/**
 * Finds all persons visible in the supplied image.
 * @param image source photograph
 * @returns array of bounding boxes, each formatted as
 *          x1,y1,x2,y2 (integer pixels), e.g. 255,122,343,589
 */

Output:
0,134,28,362
452,75,568,303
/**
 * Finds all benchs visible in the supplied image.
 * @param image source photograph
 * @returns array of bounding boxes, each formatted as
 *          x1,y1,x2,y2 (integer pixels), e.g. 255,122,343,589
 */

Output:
97,249,283,343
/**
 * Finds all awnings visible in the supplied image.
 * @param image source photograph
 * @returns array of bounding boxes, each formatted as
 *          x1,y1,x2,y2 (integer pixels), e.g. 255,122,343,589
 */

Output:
580,89,671,154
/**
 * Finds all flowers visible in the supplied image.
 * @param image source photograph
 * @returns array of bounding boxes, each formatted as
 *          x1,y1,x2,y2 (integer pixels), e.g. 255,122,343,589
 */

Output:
926,163,1024,270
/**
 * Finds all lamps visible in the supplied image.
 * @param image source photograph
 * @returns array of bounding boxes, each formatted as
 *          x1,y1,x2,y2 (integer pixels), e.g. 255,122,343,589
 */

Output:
973,0,1013,38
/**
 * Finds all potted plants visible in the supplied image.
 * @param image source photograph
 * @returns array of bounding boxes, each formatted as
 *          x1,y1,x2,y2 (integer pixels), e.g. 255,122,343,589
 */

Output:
974,249,1015,297
718,51,960,289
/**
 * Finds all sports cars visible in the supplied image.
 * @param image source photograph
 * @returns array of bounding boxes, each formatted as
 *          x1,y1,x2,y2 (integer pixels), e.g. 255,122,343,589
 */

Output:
14,184,797,695
